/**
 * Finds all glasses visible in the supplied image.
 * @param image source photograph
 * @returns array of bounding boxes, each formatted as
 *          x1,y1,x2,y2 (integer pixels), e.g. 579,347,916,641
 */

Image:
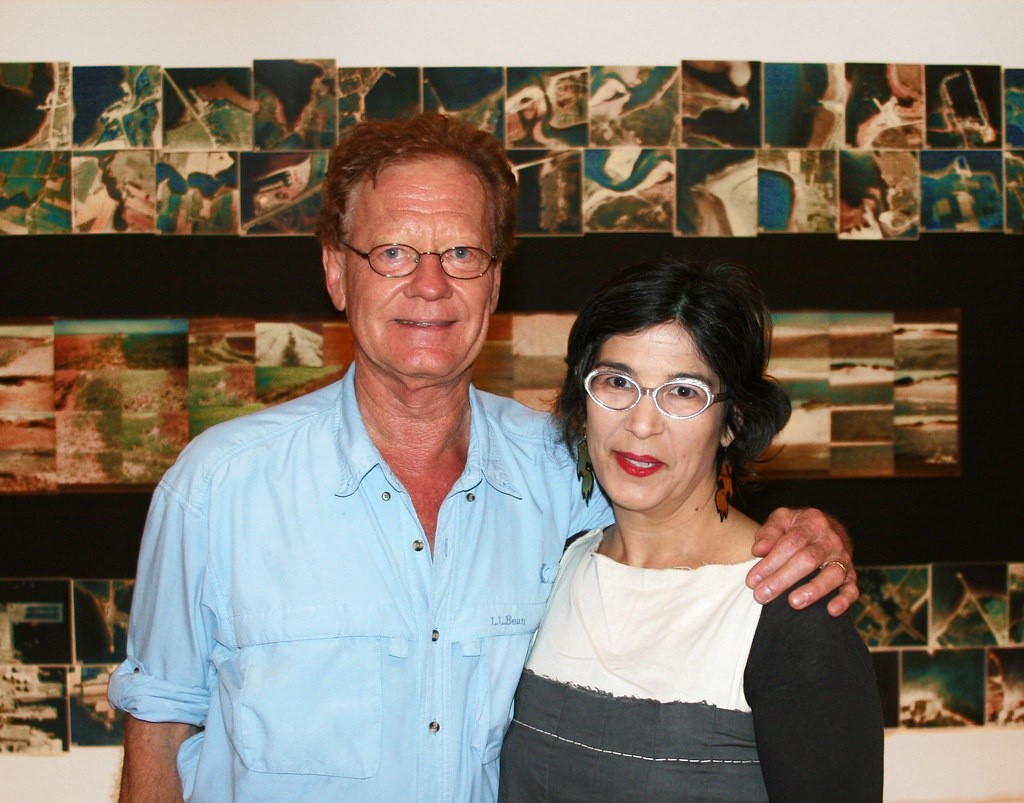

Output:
582,365,726,419
334,235,503,280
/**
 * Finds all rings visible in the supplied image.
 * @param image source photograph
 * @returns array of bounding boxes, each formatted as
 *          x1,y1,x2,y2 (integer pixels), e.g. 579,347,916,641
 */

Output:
819,555,850,580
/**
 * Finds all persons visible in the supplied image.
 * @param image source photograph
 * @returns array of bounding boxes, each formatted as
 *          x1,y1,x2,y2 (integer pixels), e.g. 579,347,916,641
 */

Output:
495,262,884,802
105,114,861,801
